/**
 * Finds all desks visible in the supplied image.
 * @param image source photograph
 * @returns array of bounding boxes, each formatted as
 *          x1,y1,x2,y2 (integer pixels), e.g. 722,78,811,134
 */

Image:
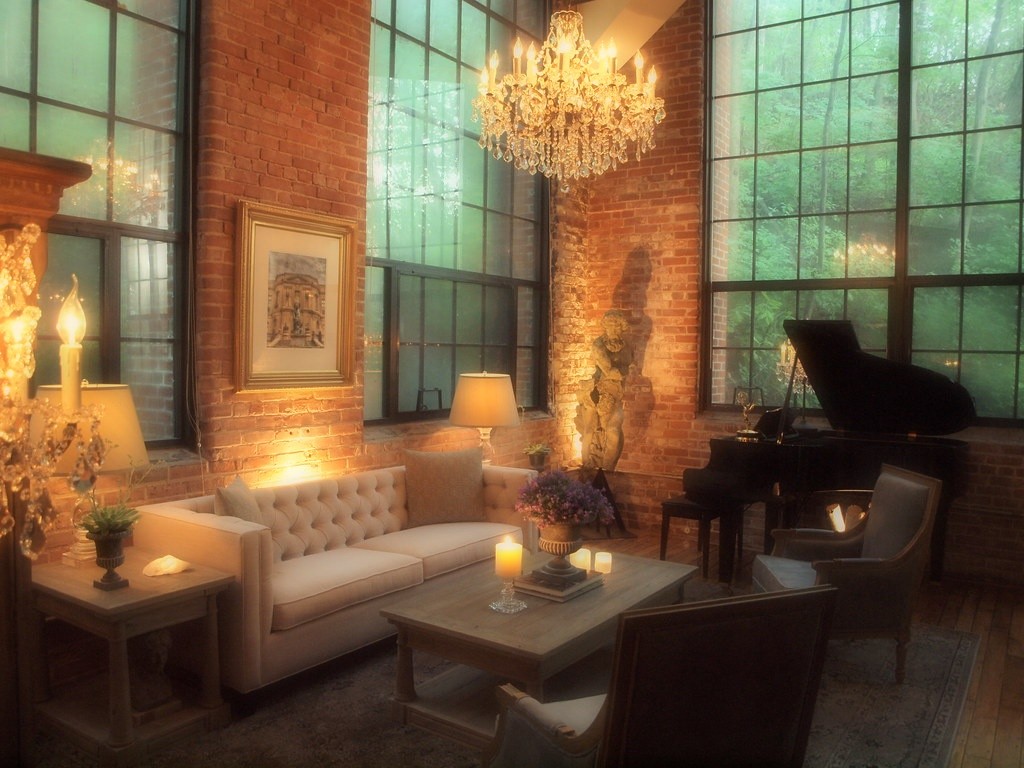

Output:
27,550,236,768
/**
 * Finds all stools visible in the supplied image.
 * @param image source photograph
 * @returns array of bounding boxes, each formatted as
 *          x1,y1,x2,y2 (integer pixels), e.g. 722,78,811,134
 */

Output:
661,497,744,581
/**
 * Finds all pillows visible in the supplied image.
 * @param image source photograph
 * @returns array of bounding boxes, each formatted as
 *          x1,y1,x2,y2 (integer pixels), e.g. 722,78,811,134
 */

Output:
216,476,264,525
400,450,489,527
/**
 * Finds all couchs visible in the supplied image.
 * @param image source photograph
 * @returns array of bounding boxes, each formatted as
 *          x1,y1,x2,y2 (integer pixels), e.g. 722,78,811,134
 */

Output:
131,466,540,719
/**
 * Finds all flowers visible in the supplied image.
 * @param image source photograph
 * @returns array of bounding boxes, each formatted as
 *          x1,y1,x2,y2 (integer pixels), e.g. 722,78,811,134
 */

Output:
513,468,620,529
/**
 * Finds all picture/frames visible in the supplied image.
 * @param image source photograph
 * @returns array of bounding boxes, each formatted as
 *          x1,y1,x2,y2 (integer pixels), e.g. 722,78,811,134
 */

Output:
234,198,358,390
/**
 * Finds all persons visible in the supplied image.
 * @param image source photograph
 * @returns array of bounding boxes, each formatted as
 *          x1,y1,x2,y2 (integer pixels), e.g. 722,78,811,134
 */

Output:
574,310,632,471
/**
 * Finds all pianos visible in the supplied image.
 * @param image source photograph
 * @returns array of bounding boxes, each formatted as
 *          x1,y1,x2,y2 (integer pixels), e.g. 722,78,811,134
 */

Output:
657,319,979,585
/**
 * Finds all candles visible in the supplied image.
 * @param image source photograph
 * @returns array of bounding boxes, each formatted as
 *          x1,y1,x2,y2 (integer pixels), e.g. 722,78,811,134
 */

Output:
570,548,591,571
595,551,612,574
493,537,524,577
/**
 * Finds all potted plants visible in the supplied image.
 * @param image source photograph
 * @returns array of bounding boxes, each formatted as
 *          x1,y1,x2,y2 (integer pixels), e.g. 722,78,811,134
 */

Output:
79,505,139,590
523,442,551,471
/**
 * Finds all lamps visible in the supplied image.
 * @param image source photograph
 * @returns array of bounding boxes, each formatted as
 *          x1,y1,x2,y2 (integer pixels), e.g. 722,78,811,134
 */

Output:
0,223,107,560
59,146,169,224
448,370,518,462
470,0,667,185
28,383,147,569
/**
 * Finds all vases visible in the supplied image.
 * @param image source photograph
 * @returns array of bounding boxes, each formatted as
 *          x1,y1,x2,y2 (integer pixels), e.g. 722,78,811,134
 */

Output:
529,522,588,588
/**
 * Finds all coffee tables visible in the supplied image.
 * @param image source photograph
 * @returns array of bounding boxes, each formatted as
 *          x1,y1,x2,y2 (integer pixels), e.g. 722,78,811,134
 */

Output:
379,550,700,768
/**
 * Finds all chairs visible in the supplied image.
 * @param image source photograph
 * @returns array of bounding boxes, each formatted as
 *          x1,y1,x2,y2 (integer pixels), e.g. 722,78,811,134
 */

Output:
481,585,836,768
754,463,941,675
